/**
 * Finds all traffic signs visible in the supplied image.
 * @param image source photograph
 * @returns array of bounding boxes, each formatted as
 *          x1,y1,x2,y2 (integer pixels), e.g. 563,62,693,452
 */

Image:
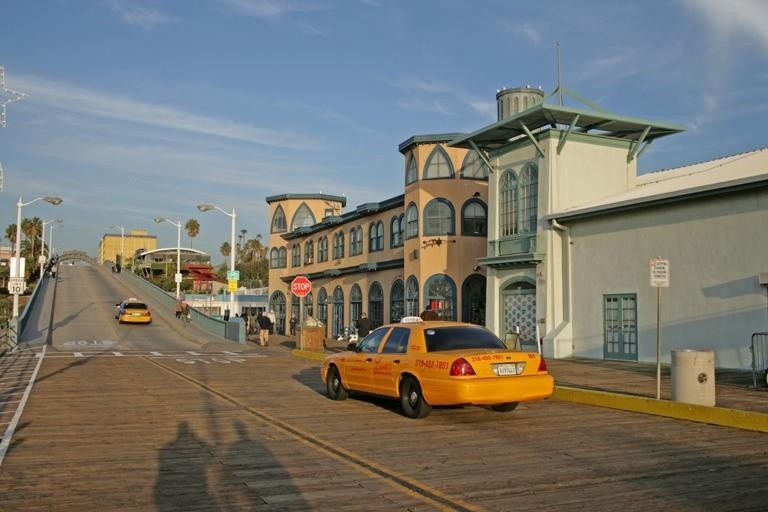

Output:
225,269,240,281
228,279,237,292
8,277,24,294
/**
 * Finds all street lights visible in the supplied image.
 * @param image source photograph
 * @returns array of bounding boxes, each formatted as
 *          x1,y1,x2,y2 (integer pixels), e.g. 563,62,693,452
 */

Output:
107,222,126,269
39,216,65,277
154,216,182,301
197,203,237,318
12,191,64,321
94,237,105,268
48,224,66,262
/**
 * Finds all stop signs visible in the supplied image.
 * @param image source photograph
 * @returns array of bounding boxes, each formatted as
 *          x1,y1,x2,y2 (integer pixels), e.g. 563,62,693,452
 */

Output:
290,276,312,298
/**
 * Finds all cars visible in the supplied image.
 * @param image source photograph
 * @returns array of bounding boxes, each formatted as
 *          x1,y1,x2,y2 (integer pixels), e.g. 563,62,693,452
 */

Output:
319,315,556,420
114,297,152,325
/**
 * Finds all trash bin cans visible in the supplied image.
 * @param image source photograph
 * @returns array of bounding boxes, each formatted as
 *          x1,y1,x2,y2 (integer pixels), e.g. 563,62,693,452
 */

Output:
670,349,716,407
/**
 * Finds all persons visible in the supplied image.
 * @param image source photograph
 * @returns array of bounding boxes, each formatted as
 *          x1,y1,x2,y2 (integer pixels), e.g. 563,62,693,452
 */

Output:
175,298,181,320
290,313,297,336
238,308,275,347
111,260,121,272
49,258,57,278
181,299,190,327
420,305,439,321
354,313,371,343
